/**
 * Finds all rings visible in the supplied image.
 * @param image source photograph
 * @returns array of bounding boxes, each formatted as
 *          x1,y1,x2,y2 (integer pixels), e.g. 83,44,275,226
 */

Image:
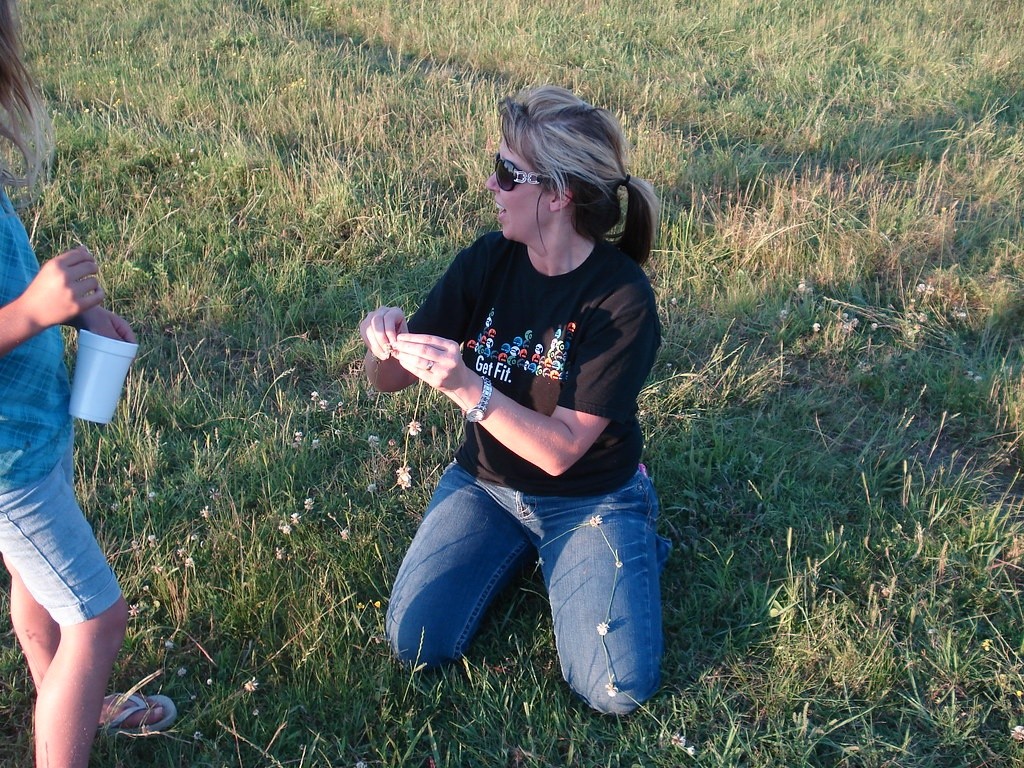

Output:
426,360,434,370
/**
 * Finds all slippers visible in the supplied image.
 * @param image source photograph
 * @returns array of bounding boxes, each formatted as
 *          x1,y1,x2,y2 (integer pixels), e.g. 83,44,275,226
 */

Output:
98,692,177,734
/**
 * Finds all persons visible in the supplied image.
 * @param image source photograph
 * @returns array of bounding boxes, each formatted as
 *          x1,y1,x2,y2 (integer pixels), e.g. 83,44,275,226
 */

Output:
0,2,176,767
365,85,664,716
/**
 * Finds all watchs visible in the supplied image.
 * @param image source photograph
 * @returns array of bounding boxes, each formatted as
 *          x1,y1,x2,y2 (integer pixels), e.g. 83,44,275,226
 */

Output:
463,375,492,423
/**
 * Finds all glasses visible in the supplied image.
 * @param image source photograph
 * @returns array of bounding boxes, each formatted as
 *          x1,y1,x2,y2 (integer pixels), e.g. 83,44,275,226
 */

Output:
494,151,559,192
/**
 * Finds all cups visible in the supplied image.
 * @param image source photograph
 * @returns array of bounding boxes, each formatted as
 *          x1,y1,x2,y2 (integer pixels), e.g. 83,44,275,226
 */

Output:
68,328,139,424
